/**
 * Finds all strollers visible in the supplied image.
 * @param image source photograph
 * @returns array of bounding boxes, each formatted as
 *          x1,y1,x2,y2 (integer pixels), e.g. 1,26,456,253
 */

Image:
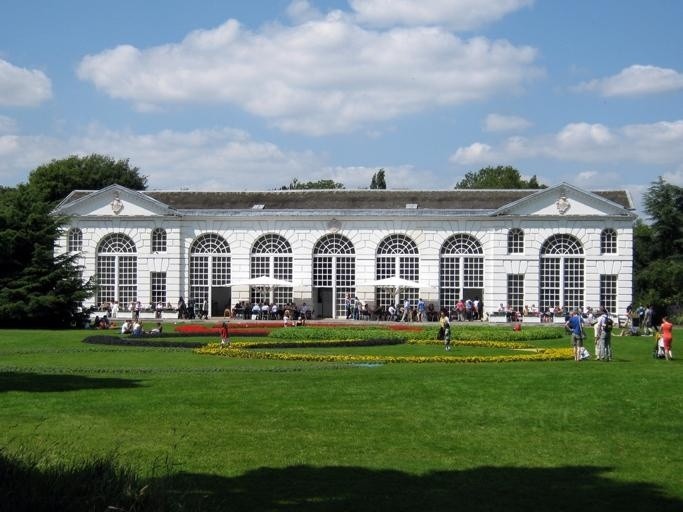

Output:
655,333,665,358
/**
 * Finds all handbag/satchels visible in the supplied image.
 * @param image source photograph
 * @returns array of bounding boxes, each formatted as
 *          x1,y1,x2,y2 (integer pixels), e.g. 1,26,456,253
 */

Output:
580,333,586,339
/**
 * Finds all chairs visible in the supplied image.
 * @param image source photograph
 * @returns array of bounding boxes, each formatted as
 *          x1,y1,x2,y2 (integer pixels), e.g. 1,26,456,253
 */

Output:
540,311,553,322
507,311,523,322
237,306,315,321
354,306,480,323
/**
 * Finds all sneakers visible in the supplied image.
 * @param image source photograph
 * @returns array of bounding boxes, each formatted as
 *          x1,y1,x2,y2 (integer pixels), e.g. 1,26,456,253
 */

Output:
595,355,612,361
655,354,674,361
445,346,452,352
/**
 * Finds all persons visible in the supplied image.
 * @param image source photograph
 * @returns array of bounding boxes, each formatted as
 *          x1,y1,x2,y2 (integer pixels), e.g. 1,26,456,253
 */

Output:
344,294,483,323
70,295,207,336
219,319,234,350
659,315,674,360
498,303,613,363
438,307,449,339
442,322,451,352
619,302,658,337
222,298,308,327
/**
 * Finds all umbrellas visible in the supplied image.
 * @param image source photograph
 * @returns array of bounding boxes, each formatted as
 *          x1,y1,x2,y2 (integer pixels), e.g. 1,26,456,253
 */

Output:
223,274,295,302
355,274,438,305
